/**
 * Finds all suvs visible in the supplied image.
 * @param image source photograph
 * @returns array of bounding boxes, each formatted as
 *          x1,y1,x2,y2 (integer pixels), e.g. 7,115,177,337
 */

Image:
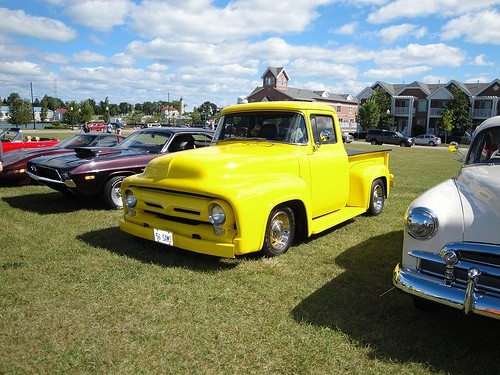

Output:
365,128,414,147
342,134,354,143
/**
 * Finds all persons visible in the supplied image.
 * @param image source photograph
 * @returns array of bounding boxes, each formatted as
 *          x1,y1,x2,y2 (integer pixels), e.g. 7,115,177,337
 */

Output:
83,125,90,133
116,119,122,135
107,122,113,134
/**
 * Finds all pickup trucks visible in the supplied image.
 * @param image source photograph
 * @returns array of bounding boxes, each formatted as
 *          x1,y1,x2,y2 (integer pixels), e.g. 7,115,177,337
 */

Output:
118,99,395,260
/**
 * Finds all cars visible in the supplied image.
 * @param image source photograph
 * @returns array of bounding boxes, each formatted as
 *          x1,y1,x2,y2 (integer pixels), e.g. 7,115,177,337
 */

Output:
0,132,146,182
88,123,107,132
414,134,441,146
23,125,216,210
392,114,500,322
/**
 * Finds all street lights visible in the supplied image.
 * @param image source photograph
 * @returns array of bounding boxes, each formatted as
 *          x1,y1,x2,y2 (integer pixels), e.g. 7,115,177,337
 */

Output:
106,106,109,125
78,108,81,129
70,106,75,130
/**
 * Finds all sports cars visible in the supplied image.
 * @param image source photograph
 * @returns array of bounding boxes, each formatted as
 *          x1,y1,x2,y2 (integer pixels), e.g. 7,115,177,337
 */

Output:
0,127,60,154
